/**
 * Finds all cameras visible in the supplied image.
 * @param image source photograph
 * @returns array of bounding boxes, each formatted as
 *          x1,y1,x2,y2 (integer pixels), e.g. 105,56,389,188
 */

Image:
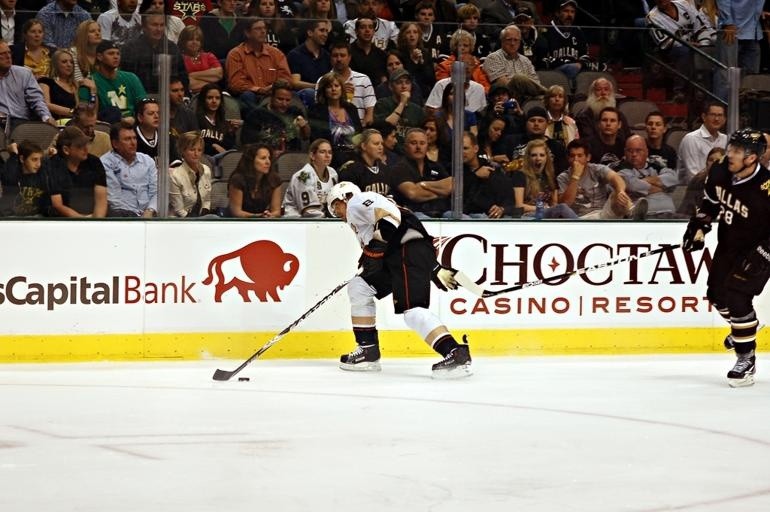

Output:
502,101,516,110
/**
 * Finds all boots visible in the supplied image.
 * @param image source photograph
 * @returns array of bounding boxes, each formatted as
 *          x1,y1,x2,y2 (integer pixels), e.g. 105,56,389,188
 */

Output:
724,320,759,379
341,330,380,365
432,335,471,370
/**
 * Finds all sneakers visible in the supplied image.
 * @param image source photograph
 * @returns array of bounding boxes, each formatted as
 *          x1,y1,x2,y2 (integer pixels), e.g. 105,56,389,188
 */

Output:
632,197,648,220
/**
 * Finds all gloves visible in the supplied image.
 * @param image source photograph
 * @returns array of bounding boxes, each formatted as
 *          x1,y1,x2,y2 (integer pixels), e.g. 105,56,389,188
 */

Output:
683,218,711,252
359,245,384,271
432,265,461,292
738,243,769,276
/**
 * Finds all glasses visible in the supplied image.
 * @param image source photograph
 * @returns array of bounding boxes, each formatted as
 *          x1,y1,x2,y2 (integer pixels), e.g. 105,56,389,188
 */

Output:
140,98,159,103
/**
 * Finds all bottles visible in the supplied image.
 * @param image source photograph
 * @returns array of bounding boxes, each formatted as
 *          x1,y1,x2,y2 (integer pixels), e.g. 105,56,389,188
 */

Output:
345,78,354,103
535,197,544,218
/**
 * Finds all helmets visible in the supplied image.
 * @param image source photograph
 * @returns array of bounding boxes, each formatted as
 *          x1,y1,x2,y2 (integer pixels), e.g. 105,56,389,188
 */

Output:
731,128,766,154
327,181,361,216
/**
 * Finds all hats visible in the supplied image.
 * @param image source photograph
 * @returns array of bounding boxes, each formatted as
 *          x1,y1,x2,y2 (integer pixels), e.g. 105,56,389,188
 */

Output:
527,106,548,119
389,69,411,82
557,0,578,8
58,126,92,146
94,40,119,66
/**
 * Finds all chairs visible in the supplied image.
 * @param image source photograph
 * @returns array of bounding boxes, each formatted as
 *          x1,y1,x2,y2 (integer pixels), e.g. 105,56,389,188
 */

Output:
221,95,243,145
541,70,618,97
615,101,696,151
276,150,311,191
8,117,59,152
203,149,243,207
95,120,112,135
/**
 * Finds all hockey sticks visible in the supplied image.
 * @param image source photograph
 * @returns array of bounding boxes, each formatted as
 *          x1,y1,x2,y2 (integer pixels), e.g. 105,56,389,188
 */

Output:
451,245,683,298
213,271,363,382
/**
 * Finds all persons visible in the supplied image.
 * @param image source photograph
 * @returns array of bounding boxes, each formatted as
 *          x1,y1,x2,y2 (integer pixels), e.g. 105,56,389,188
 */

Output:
682,124,770,380
325,180,472,370
